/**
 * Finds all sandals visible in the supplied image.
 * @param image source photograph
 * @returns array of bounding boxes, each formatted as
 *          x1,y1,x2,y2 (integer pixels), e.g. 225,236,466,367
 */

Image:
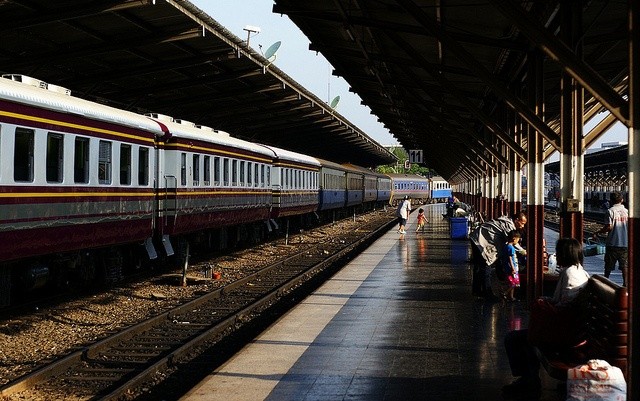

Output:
499,292,508,304
510,298,520,303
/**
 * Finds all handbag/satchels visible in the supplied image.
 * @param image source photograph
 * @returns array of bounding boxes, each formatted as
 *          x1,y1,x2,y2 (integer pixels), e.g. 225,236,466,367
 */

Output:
547,318,601,366
527,297,567,336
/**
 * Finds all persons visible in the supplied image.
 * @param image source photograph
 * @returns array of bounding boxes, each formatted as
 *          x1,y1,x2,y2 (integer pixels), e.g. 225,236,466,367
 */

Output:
396,195,412,235
593,185,628,286
415,207,430,233
453,205,467,217
451,195,461,203
471,213,528,301
500,229,521,300
502,238,591,389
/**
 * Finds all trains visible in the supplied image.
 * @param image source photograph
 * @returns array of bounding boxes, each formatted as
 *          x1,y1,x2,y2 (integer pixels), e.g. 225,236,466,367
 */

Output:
1,73,452,313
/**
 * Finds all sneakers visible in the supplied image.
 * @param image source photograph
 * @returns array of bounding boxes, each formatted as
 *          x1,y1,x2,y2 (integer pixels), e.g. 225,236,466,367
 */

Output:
415,229,418,234
397,229,406,234
442,214,447,218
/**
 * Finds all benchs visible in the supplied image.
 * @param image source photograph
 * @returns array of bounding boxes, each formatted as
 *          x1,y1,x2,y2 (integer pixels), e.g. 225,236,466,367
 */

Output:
531,275,629,395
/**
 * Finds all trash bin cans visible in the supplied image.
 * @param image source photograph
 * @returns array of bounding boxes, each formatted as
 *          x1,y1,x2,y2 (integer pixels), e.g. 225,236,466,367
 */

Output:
451,243,470,262
449,217,468,240
